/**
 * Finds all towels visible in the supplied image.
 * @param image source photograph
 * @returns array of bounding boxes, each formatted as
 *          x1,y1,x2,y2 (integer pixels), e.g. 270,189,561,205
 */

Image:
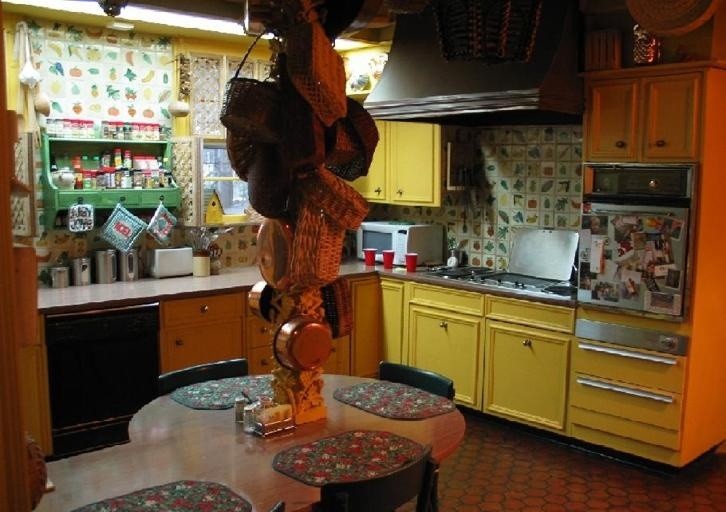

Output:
2,19,41,146
321,278,355,338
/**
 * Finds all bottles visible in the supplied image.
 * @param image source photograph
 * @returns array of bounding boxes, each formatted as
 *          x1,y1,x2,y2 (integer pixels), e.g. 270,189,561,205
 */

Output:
50,150,57,171
62,151,71,171
446,249,458,267
47,266,69,288
46,118,162,141
73,149,169,191
71,256,92,286
243,408,255,433
209,258,221,275
633,22,663,66
232,396,245,423
119,248,139,282
93,246,116,284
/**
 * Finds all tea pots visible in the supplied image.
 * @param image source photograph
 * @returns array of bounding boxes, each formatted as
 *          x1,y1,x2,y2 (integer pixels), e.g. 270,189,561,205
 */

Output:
53,169,75,189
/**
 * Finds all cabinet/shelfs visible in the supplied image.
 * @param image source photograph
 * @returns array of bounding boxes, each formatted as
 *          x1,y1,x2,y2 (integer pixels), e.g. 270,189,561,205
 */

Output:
377,274,404,366
1,2,51,511
335,121,475,208
578,59,726,162
244,290,350,374
402,274,578,454
160,286,248,376
41,126,181,230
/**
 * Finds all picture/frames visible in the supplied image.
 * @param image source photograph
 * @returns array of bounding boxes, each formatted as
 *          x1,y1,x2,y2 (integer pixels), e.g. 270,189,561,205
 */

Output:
446,142,474,190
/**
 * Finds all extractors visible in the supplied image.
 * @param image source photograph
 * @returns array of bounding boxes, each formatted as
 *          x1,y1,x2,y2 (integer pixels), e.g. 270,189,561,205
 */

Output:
362,1,585,125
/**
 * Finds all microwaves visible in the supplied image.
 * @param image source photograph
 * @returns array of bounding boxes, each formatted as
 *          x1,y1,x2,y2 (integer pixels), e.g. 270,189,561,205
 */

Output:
356,219,445,266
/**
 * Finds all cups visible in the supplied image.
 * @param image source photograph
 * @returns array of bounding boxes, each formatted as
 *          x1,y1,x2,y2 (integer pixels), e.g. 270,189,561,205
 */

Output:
382,249,395,270
405,253,418,273
364,248,378,265
193,256,211,277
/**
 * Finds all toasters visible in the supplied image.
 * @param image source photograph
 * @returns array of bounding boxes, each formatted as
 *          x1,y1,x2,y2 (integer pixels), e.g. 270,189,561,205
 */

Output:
148,246,194,277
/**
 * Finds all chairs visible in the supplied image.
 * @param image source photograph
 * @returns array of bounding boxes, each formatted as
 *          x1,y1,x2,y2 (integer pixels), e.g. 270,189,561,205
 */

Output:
310,444,439,512
158,358,248,397
349,273,380,379
270,502,286,512
379,361,455,402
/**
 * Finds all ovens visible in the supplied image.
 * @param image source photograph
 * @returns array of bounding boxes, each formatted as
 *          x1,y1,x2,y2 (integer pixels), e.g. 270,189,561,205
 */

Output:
575,162,691,322
42,302,161,460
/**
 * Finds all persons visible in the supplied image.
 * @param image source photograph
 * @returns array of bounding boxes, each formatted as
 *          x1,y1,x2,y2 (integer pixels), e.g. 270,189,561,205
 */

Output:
594,277,637,301
586,216,603,235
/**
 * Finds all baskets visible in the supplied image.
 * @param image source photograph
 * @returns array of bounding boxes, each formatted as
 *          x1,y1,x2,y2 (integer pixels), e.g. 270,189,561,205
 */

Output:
219,16,379,338
217,16,379,337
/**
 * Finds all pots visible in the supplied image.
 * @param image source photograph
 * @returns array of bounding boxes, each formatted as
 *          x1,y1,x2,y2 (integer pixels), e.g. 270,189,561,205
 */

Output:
269,313,333,370
246,282,276,322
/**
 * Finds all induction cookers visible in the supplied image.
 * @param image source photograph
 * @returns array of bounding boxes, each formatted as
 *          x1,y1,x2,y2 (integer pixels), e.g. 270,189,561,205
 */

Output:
435,265,556,295
